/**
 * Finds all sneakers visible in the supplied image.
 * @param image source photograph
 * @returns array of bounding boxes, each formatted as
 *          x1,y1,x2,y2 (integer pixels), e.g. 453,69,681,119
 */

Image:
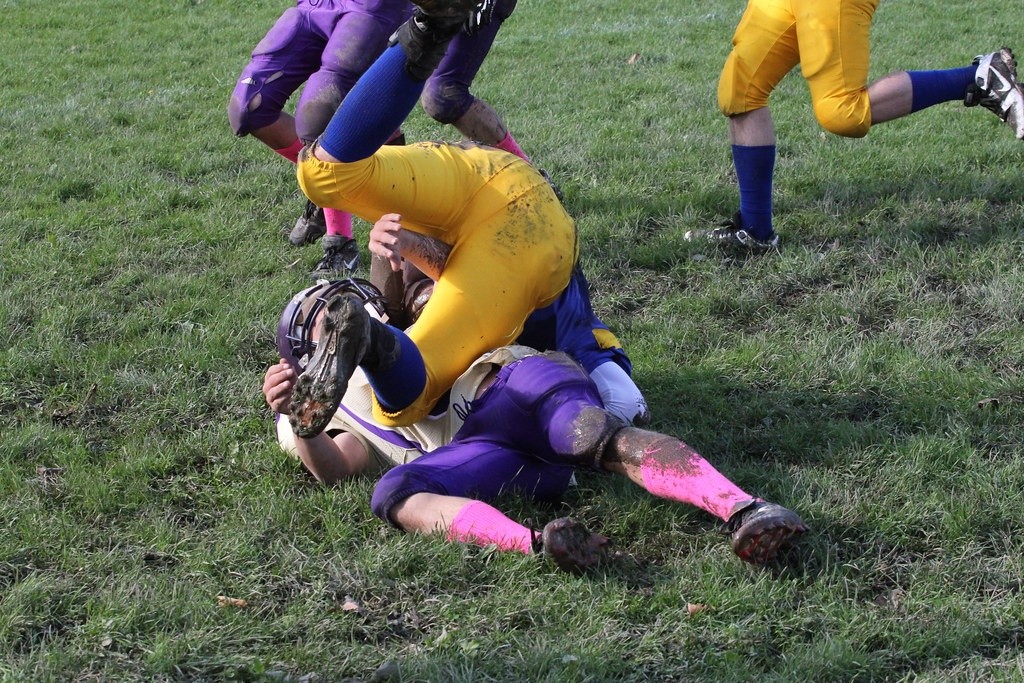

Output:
315,234,358,280
539,168,562,200
963,46,1024,139
530,516,614,563
386,0,484,83
288,293,371,440
717,498,809,560
289,198,326,246
683,210,778,255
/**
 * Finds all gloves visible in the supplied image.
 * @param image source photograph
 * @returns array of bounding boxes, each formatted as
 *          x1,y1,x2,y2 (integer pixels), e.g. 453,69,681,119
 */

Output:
462,0,518,36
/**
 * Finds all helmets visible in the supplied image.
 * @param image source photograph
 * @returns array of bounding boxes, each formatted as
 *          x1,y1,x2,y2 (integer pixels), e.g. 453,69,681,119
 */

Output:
278,277,399,375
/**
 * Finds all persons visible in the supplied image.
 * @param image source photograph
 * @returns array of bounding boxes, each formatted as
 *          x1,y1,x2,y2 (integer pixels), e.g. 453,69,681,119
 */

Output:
685,0,1024,248
230,0,811,574
